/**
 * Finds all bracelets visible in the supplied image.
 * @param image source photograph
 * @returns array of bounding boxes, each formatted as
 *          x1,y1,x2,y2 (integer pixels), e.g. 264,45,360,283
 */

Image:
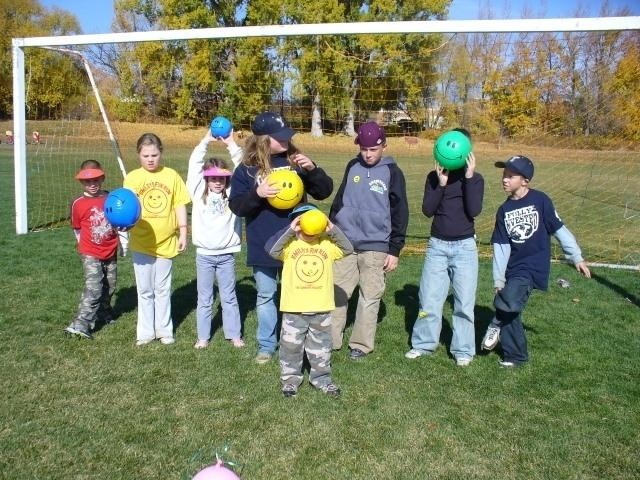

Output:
179,225,187,229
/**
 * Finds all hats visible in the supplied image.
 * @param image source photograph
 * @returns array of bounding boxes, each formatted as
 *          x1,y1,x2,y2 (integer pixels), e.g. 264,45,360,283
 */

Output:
203,167,232,177
354,122,385,147
494,155,534,181
75,169,104,179
251,112,296,141
288,205,317,220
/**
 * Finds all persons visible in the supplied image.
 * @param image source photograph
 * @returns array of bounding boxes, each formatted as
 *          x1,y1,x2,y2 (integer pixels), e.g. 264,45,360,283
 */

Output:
263,202,354,398
113,133,191,346
64,159,129,341
480,155,591,367
184,126,246,349
228,111,333,364
404,128,485,366
327,121,409,361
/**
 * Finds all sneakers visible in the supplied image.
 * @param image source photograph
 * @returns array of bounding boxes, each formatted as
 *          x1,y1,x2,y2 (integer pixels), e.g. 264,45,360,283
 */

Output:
256,352,271,362
322,383,340,395
405,350,420,358
232,338,244,347
457,358,470,366
137,337,174,345
283,383,297,396
195,340,209,349
66,325,93,339
351,349,365,358
480,323,501,351
